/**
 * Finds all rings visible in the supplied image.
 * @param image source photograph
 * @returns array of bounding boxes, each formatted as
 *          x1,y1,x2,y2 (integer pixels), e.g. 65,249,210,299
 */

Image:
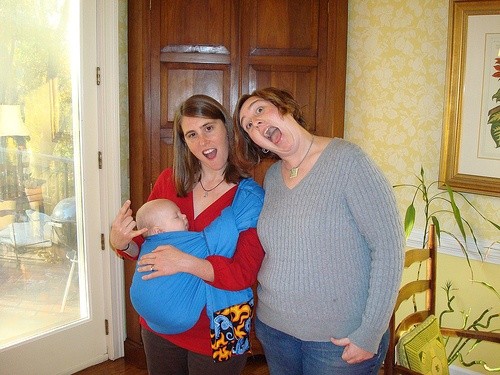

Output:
151,264,155,272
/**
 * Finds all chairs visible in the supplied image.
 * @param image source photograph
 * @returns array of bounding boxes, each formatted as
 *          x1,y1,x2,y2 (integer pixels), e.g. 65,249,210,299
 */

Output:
383,223,500,375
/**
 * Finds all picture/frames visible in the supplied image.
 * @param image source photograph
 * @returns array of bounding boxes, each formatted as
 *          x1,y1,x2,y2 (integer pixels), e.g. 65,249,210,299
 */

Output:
438,1,500,198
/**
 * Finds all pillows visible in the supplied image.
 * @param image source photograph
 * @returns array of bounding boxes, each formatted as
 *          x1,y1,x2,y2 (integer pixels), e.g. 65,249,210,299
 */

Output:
398,314,450,375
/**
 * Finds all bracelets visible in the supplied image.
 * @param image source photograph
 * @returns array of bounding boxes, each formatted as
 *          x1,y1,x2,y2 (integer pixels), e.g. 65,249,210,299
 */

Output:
119,243,130,251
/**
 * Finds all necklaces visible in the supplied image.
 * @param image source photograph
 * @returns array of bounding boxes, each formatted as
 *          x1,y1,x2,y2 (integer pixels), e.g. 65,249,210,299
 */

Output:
200,178,226,197
282,134,314,178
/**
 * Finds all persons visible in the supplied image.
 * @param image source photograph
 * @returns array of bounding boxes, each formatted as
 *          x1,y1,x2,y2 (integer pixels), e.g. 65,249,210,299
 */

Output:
232,86,407,375
109,94,266,375
130,198,208,335
